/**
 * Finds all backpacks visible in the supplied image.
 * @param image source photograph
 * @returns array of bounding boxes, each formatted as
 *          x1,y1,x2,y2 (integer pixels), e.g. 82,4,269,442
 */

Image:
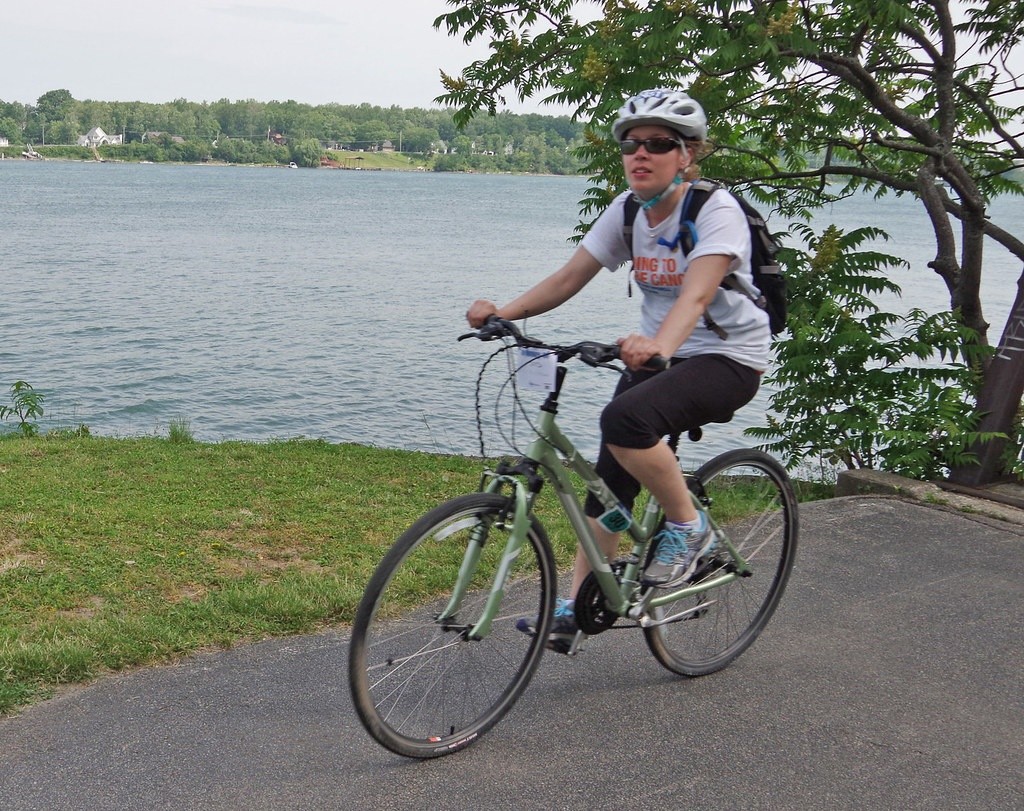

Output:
623,177,787,341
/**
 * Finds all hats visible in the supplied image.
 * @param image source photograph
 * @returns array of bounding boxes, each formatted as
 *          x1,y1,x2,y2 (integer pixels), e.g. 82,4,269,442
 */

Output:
611,88,707,149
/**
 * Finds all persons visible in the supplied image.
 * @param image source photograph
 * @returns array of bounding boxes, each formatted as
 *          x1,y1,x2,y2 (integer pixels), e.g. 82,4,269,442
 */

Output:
466,89,771,652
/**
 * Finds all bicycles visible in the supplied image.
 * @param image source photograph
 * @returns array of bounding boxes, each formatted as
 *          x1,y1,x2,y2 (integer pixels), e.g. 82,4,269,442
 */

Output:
348,313,798,761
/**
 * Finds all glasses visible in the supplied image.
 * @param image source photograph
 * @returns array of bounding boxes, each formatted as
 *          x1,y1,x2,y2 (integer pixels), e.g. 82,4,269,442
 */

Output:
620,138,687,155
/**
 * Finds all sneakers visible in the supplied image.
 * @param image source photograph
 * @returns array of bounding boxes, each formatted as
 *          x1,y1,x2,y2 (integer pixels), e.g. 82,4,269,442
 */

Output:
516,598,589,655
641,510,714,589
